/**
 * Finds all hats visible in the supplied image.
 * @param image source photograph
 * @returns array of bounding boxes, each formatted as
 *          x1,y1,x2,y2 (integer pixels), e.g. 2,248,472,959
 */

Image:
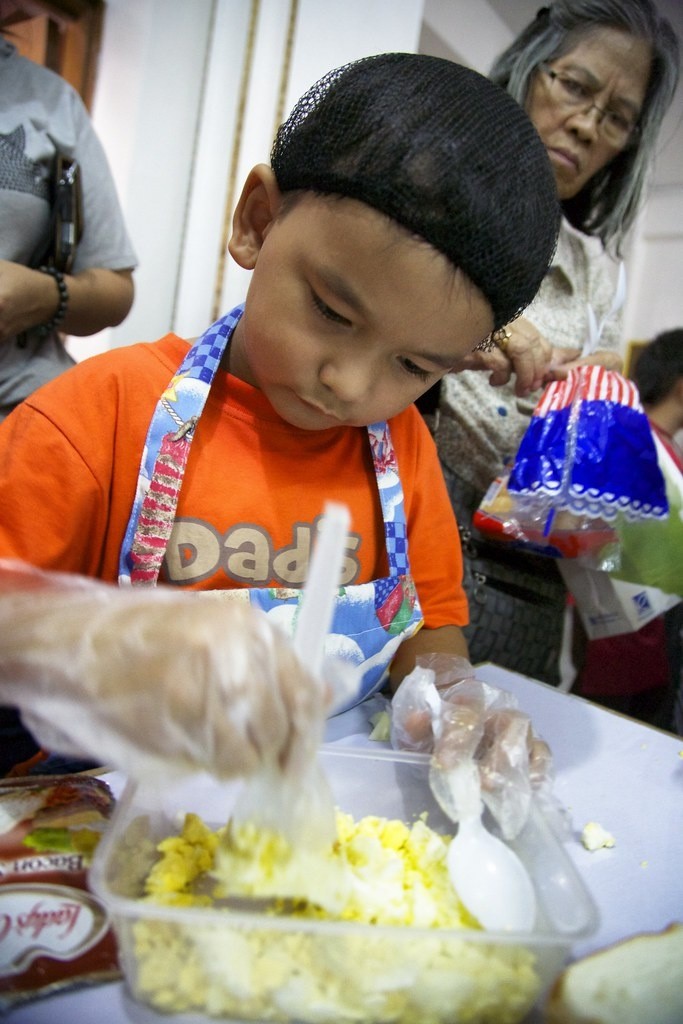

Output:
271,52,561,333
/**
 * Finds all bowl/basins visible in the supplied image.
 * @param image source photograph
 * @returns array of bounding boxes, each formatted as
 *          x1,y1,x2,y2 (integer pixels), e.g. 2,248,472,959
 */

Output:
87,738,604,1024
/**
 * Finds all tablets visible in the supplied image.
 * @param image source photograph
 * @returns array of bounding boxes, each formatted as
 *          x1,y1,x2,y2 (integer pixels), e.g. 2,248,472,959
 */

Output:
56,169,74,274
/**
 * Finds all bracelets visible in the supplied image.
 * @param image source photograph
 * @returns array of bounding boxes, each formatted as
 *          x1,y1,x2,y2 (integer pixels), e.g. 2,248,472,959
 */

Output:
35,266,69,336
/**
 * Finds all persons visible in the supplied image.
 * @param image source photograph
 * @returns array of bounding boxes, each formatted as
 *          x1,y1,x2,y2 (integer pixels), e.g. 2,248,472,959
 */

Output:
415,0,683,741
0,35,142,422
0,52,563,794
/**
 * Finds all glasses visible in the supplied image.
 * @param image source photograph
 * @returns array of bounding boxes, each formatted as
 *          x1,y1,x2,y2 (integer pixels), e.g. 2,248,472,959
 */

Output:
538,61,640,145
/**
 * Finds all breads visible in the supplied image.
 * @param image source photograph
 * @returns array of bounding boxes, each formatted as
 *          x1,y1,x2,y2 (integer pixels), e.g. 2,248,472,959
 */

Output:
547,920,683,1024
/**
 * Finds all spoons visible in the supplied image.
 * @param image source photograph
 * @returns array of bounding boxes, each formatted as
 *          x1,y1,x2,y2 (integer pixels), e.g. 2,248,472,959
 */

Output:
427,681,540,938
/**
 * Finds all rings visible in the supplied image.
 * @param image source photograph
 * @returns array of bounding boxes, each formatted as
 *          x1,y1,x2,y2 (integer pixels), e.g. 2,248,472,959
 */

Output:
499,330,515,351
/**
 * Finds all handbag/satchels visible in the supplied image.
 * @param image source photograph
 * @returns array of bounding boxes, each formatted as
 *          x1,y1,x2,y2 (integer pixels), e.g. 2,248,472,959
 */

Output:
51,153,80,273
474,364,683,600
462,531,566,686
